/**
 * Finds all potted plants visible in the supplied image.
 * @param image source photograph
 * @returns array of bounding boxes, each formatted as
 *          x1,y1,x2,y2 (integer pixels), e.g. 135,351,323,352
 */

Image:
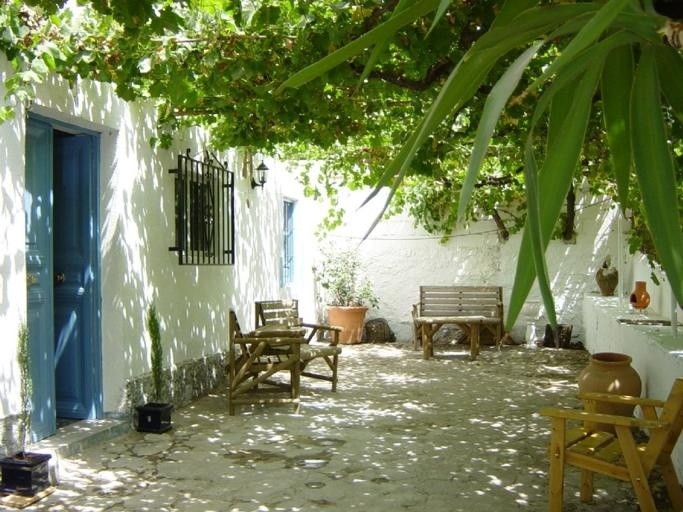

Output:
0,312,52,496
134,300,175,432
314,244,380,344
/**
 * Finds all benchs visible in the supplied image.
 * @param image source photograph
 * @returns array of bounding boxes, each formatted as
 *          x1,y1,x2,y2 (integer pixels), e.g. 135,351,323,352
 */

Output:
411,285,502,350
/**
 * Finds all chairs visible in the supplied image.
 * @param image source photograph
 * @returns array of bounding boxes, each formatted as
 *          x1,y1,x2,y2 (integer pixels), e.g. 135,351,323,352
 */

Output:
546,378,683,511
230,299,342,414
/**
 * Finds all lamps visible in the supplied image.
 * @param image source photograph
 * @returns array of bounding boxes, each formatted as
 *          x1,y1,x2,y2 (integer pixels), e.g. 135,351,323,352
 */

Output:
251,159,266,189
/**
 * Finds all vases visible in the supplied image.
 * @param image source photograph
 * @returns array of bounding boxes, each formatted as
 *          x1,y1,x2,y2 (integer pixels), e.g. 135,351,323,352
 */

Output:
597,259,650,310
580,350,639,428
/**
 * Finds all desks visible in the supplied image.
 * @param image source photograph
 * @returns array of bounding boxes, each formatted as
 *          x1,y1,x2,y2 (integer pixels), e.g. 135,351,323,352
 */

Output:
415,313,483,357
